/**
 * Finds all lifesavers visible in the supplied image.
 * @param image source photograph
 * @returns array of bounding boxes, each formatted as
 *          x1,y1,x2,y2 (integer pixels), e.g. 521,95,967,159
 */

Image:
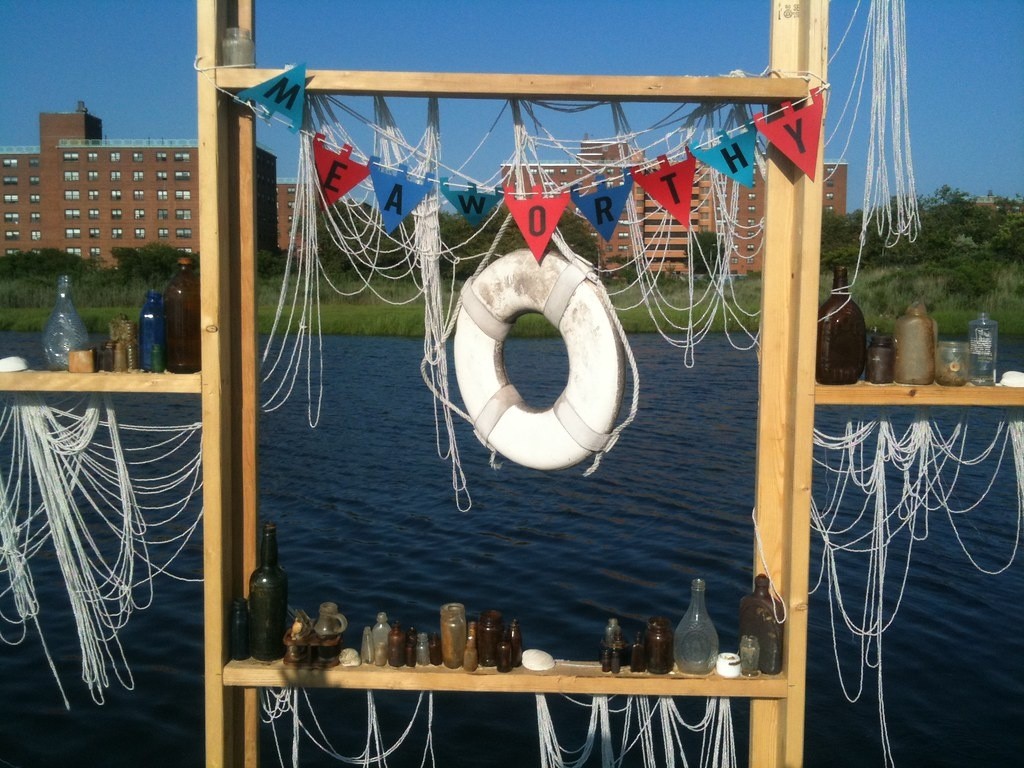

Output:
453,249,627,470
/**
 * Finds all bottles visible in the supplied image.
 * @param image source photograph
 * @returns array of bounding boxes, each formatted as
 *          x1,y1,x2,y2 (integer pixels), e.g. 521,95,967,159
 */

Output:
602,579,720,675
739,574,784,676
151,344,164,371
136,290,167,372
361,603,524,673
249,523,287,660
163,257,201,373
41,275,90,371
967,309,998,386
97,339,140,373
816,267,936,383
230,598,250,660
935,341,971,386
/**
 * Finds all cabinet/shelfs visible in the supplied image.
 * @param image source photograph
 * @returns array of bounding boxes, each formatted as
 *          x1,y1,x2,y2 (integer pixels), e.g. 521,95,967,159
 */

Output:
0,0,1024,767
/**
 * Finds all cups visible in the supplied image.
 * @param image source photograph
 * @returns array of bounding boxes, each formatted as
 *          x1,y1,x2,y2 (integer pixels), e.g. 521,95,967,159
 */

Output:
317,602,347,635
716,652,741,678
740,634,760,677
